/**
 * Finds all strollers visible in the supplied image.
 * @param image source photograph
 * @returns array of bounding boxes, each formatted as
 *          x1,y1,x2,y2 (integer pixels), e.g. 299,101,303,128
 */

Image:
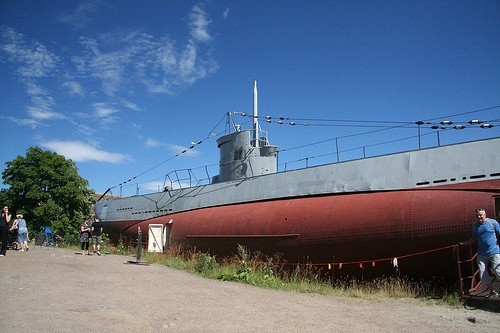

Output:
0,228,19,250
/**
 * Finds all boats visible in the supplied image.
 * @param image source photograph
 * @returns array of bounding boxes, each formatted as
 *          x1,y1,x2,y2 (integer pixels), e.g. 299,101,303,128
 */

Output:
91,80,500,285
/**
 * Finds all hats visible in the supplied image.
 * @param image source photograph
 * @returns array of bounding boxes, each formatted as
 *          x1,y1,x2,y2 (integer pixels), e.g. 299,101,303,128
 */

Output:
16,214,23,218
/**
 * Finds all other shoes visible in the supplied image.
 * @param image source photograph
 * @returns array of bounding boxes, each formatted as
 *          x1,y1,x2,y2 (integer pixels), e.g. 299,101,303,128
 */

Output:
94,251,102,256
17,246,29,251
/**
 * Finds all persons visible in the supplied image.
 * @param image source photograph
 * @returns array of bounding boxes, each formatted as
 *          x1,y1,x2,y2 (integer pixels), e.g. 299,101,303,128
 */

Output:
0,204,30,257
471,208,500,298
80,215,103,256
